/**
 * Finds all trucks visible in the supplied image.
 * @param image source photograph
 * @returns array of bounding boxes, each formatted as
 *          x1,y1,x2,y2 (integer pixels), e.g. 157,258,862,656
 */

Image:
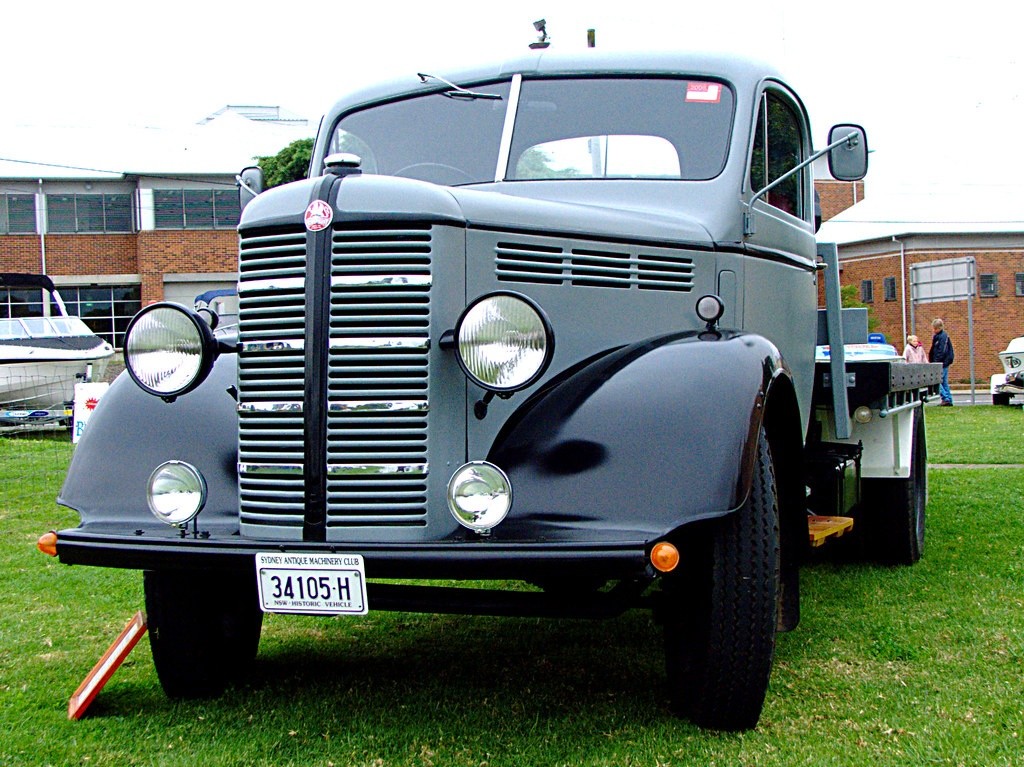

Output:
992,336,1024,404
37,43,941,734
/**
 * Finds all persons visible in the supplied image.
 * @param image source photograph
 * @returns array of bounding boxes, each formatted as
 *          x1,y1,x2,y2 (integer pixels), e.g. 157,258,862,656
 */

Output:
929,319,954,406
902,335,928,363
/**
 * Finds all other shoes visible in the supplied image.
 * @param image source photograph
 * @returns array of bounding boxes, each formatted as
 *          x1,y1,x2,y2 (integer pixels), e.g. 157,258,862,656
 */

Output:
937,401,953,407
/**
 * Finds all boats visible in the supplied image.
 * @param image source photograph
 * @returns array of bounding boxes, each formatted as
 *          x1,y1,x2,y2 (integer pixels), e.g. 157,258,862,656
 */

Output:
0,273,115,409
